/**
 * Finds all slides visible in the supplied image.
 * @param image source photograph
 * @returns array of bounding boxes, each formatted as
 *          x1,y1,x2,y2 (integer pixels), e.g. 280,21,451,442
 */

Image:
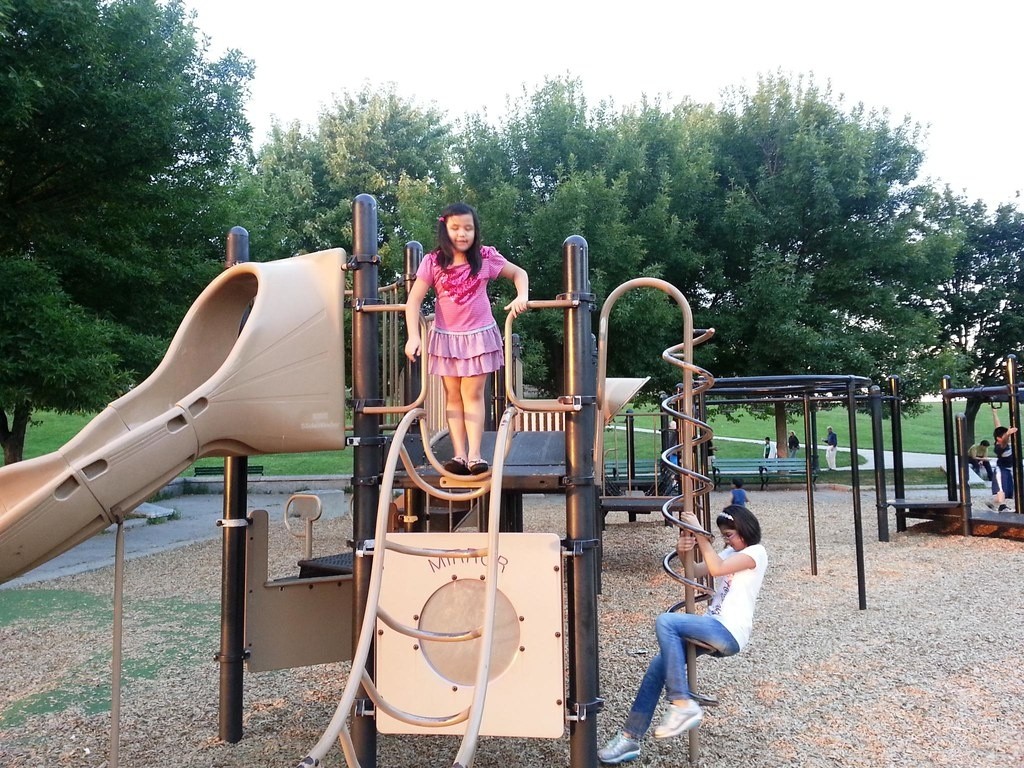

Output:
0,248,346,585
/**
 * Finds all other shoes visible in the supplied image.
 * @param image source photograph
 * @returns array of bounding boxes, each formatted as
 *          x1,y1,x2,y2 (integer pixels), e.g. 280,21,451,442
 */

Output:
998,506,1017,513
467,458,489,474
445,457,471,475
985,501,999,513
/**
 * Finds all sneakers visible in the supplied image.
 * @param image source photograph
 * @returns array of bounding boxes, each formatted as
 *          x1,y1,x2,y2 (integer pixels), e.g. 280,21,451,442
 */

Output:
598,728,641,763
654,699,705,740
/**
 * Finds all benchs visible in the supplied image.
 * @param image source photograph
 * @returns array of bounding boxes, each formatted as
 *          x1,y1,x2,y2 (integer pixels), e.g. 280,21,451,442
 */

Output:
193,465,264,476
602,460,678,486
712,456,818,491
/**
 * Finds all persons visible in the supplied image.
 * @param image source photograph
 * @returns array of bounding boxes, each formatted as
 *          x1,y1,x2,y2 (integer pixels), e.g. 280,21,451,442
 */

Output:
668,421,678,455
788,430,799,457
708,441,718,465
405,206,528,475
596,505,768,763
968,440,993,481
985,406,1018,513
729,478,751,508
763,437,776,458
821,426,837,469
